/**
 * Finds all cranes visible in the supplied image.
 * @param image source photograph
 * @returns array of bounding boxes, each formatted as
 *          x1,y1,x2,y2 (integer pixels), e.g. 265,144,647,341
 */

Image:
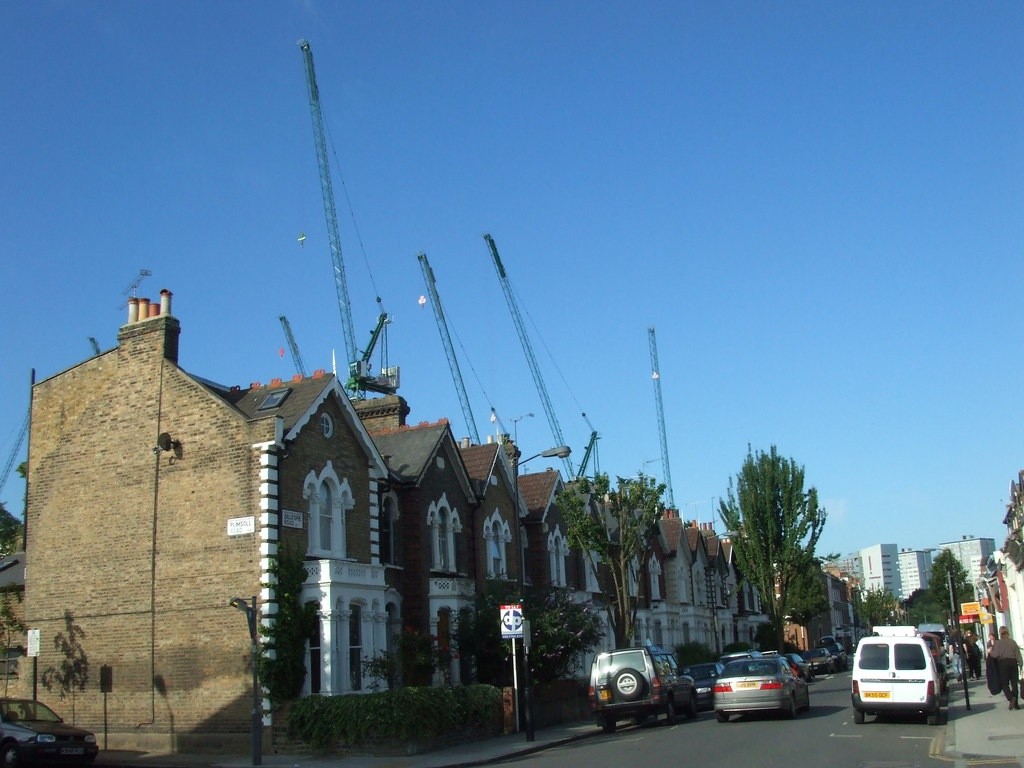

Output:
275,38,687,520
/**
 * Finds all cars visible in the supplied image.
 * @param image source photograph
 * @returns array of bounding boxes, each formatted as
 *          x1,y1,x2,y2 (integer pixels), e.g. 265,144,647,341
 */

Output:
714,656,811,724
0,696,100,768
680,663,718,712
802,649,836,675
784,653,813,683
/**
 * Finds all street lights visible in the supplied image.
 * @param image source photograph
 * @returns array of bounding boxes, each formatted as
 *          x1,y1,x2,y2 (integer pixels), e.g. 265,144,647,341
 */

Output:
509,445,572,742
228,594,264,767
923,547,971,711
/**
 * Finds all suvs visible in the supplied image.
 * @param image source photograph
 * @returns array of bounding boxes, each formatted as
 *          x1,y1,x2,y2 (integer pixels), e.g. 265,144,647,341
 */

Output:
588,645,697,734
823,643,849,671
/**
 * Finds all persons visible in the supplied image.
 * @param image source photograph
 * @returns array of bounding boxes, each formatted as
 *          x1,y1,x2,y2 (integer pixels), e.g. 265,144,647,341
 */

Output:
991,625,1023,710
986,633,998,653
948,626,984,682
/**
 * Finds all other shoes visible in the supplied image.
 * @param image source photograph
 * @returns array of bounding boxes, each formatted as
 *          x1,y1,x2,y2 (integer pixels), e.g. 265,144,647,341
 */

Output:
970,677,974,681
1008,697,1016,710
973,675,977,680
1014,702,1021,710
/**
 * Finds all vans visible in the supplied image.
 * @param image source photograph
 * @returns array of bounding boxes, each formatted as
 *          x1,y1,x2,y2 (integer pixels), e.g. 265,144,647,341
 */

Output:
920,632,948,675
851,626,946,726
